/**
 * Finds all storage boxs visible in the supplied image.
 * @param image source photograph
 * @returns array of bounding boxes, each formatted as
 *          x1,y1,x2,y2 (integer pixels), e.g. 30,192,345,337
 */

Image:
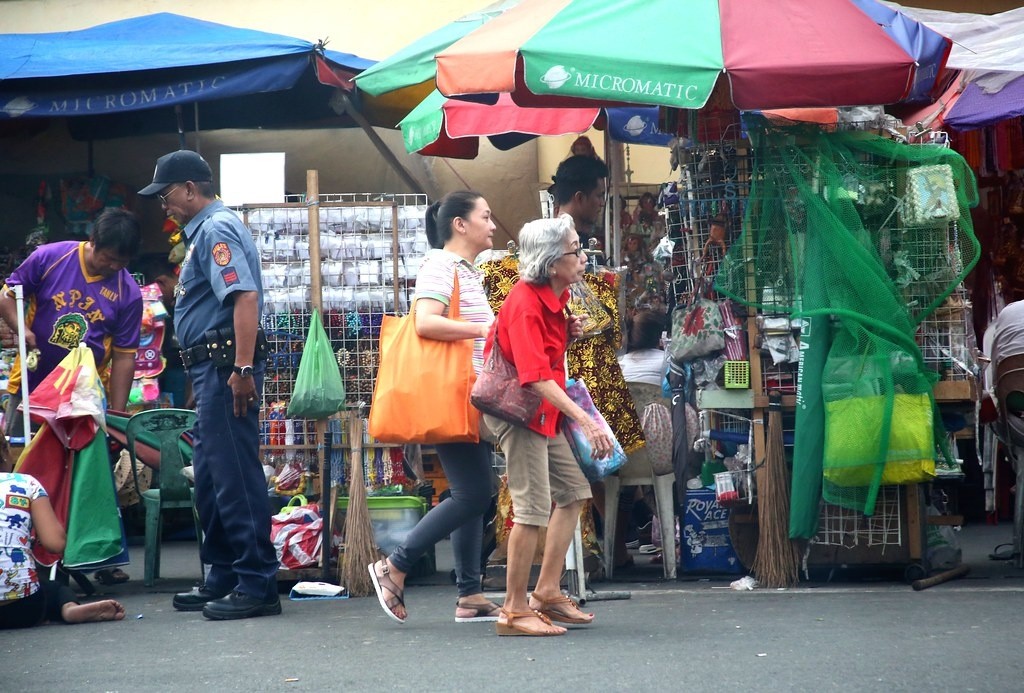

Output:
627,546,663,566
328,496,436,585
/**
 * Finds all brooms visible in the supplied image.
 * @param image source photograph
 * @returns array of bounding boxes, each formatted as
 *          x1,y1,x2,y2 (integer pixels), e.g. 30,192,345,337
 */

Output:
752,388,800,593
334,416,386,599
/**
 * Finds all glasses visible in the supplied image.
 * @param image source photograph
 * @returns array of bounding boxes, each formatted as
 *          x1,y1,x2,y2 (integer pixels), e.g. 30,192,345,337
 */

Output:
160,182,198,203
563,242,584,257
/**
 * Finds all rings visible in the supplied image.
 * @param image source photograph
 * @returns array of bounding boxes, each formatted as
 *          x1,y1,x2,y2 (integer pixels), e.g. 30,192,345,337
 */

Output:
248,397,253,401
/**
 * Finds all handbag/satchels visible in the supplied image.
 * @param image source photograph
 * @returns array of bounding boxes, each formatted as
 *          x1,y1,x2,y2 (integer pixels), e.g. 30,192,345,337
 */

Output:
822,334,935,486
671,298,726,361
469,303,575,428
926,504,962,571
559,378,628,483
287,307,346,419
367,267,480,445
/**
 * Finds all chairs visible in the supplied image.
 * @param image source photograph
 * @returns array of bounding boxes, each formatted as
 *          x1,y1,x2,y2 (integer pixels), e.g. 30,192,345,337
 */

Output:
126,408,214,586
596,381,698,580
982,353,1024,569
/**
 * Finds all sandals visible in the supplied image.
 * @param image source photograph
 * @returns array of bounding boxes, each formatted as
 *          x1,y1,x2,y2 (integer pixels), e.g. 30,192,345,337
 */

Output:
454,601,503,622
368,559,405,624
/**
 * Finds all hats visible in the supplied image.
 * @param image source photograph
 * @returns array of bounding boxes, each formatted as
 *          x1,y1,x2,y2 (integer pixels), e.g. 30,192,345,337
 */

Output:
137,150,213,199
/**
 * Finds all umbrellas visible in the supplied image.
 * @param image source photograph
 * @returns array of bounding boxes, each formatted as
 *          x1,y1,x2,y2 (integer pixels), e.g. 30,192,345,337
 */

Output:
348,0,952,160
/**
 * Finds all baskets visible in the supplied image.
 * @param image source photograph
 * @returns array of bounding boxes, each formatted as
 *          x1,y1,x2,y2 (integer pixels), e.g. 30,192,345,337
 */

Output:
724,360,750,389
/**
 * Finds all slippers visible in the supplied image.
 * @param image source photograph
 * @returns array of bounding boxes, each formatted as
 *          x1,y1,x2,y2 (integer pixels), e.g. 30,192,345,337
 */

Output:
989,544,1012,559
93,569,129,584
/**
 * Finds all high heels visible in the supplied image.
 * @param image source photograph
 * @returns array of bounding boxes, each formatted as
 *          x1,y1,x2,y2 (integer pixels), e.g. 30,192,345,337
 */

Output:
531,592,592,623
496,608,554,636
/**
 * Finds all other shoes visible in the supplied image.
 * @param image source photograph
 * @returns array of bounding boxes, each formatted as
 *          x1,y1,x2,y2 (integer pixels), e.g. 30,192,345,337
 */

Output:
639,533,663,554
624,531,642,548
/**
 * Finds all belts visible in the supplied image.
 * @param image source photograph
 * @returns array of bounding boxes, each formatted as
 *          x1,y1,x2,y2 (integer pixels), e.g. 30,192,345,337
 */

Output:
178,343,212,370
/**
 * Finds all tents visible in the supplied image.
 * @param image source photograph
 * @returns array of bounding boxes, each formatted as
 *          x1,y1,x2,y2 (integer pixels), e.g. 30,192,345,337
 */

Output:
0,12,378,176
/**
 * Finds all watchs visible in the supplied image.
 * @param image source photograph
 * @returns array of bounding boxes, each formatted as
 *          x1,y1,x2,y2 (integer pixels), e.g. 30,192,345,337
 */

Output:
233,365,253,377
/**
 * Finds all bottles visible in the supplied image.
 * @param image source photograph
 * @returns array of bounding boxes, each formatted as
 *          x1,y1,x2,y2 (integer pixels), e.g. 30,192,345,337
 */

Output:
701,459,725,488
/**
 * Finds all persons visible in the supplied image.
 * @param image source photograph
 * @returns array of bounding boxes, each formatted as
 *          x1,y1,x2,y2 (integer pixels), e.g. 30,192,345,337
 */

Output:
375,190,503,624
0,429,125,630
137,150,281,619
483,217,614,635
571,136,595,156
990,299,1024,418
0,208,143,414
610,192,658,283
617,309,700,476
554,154,609,265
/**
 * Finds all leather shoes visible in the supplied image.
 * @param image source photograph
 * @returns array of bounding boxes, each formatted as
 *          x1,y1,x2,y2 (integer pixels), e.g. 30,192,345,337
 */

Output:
203,591,282,620
173,585,234,611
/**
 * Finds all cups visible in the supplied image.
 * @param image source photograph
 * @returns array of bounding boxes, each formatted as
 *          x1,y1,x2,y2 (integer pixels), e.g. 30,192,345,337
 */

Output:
716,472,734,496
